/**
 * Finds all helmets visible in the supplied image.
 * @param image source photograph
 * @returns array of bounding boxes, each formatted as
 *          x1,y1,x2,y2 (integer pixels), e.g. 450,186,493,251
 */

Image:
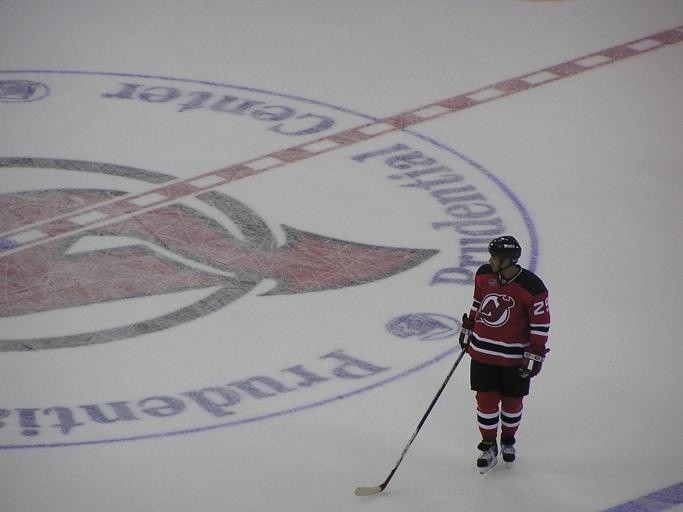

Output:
488,236,521,259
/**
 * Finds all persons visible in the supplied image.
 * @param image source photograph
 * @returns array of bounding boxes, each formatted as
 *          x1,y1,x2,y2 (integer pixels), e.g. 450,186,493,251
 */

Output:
458,235,551,467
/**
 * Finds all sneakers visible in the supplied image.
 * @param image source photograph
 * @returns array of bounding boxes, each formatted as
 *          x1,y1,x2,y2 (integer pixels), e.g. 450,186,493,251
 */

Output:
500,434,515,463
476,440,498,467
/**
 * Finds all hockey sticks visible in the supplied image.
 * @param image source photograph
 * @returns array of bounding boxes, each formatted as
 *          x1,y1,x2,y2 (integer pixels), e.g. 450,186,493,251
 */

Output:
353,345,466,496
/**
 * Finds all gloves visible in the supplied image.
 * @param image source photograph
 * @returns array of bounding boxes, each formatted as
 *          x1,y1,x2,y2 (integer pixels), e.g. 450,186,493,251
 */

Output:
458,313,475,353
518,346,550,379
